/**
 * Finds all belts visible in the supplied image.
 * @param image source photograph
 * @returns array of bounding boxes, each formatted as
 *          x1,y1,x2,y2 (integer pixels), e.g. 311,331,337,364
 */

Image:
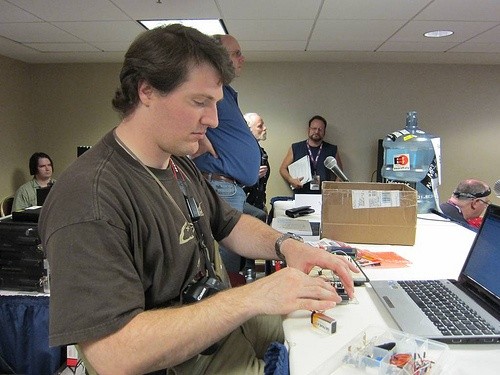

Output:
201,174,241,185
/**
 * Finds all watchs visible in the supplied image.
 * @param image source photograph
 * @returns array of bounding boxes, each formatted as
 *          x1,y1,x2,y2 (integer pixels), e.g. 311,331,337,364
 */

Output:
275,232,304,261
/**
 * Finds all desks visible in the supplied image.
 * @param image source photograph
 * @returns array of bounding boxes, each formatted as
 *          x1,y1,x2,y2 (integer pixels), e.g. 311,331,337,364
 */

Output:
273,194,500,375
0,289,63,375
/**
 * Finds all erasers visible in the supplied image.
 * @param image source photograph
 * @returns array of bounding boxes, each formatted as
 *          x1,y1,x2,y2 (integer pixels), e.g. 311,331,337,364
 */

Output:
311,312,336,333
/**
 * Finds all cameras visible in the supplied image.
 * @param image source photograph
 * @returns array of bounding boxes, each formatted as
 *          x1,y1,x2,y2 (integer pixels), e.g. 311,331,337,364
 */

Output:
182,274,225,304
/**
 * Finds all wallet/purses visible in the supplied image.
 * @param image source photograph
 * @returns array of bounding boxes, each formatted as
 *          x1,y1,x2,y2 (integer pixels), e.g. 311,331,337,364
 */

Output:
286,205,315,219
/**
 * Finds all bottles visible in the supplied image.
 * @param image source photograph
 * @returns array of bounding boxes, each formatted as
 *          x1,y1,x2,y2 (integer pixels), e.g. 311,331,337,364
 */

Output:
381,111,441,182
383,177,437,214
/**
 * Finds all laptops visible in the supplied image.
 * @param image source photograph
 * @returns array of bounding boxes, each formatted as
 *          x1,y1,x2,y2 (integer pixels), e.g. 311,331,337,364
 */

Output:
369,203,500,344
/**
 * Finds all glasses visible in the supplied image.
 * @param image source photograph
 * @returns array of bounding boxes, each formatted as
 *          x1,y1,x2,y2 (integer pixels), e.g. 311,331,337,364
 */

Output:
226,50,243,57
480,197,492,206
309,126,325,131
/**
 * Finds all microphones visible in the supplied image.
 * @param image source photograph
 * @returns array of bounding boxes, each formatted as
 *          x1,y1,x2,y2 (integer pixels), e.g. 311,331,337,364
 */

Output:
261,154,268,180
324,156,350,182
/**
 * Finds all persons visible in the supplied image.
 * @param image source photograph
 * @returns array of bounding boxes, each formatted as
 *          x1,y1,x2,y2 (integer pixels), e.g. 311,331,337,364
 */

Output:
428,179,492,225
38,23,361,375
280,115,343,194
192,35,262,274
238,113,270,215
11,152,56,212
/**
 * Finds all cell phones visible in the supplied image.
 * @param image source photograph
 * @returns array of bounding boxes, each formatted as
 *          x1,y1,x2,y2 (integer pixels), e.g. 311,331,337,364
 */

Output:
327,246,356,256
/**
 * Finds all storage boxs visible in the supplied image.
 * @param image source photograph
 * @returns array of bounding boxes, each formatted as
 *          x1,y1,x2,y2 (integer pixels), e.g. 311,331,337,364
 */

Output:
319,181,418,246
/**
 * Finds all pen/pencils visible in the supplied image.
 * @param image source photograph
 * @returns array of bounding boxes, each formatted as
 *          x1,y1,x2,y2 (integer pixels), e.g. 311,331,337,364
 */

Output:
360,259,381,264
361,263,381,268
